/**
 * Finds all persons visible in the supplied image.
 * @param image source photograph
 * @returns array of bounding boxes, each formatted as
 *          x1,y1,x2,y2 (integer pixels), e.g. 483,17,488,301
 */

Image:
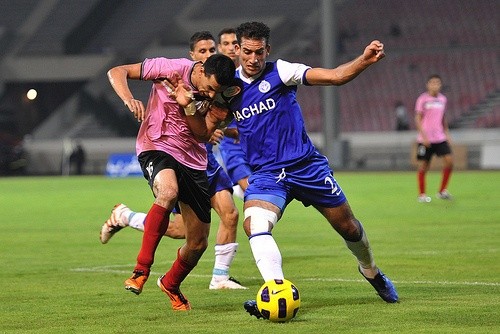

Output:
107,53,236,312
163,22,398,320
100,31,249,289
413,75,455,204
217,29,251,200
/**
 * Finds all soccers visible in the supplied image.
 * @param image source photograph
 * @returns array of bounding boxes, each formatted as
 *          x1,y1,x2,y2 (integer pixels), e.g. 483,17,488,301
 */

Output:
256,279,300,323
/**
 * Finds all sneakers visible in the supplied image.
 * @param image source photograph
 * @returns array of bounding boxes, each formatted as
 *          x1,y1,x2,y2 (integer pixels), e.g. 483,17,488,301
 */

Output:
157,274,191,311
124,270,148,295
244,300,267,320
100,202,128,244
208,275,249,290
359,263,398,303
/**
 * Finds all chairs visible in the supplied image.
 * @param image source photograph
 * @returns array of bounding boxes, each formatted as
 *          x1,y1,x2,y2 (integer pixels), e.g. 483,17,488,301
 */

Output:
297,0,500,132
